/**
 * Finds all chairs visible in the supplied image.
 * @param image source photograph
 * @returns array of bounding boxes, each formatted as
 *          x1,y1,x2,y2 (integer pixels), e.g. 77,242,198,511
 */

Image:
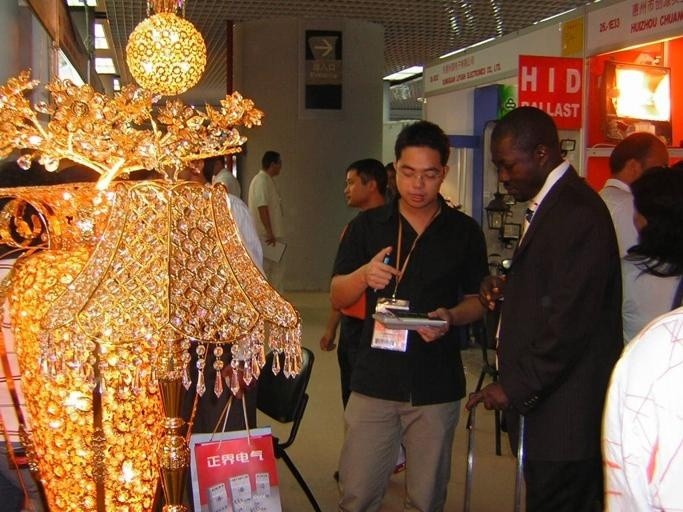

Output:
248,344,323,512
467,301,506,456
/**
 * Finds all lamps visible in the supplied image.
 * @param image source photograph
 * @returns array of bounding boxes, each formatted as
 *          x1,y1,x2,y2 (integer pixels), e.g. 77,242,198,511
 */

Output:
560,138,575,158
0,0,307,512
484,189,521,250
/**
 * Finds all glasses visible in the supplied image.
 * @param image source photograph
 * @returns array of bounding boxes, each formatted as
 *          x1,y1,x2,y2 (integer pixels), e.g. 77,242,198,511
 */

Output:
394,164,446,182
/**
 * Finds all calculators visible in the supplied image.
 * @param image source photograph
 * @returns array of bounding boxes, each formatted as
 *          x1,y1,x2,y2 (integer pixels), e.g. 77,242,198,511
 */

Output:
371,311,448,331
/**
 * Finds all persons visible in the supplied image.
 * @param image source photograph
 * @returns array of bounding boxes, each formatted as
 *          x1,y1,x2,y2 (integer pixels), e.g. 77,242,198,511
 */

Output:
158,157,263,433
212,155,241,199
0,256,45,512
248,150,286,290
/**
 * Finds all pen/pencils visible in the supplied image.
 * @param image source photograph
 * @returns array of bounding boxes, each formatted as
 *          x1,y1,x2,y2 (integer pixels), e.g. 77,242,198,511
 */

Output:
374,253,390,293
